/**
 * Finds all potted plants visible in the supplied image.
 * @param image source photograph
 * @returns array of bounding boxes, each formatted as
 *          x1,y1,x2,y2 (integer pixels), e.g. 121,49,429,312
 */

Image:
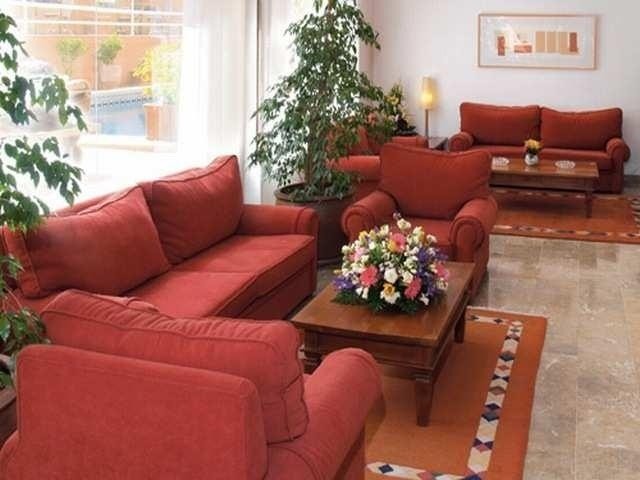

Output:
0,11,82,441
132,41,183,142
251,2,395,262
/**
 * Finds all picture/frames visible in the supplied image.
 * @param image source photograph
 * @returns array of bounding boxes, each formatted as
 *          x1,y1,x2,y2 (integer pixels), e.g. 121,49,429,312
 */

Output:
477,11,597,70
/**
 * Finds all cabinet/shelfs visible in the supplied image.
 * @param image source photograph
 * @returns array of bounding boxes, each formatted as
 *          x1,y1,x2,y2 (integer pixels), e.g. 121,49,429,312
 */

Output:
429,136,448,150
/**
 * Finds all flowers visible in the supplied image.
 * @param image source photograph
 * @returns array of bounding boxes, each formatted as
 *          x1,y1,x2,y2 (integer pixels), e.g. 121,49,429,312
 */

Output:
523,137,543,151
331,225,440,314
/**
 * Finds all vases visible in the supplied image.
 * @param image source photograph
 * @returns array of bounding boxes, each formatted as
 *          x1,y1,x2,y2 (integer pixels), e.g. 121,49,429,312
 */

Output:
524,151,540,166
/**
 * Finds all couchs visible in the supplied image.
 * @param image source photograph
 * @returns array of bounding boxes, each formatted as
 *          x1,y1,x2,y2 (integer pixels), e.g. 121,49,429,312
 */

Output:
0,284,382,480
1,151,317,318
341,139,498,299
322,111,429,198
447,99,632,197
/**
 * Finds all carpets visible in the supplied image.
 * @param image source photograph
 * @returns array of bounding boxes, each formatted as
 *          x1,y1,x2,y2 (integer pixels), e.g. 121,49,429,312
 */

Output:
487,188,640,244
277,292,546,480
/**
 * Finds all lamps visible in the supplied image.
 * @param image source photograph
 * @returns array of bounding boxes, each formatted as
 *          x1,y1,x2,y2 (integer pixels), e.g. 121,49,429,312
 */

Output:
420,77,439,137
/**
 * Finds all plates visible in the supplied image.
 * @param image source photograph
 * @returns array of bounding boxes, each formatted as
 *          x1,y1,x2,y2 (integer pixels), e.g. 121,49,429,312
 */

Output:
492,157,509,165
555,160,576,170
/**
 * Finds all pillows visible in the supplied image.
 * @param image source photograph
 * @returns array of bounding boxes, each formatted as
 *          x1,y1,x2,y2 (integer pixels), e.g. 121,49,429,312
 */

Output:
40,288,304,444
140,151,245,245
0,186,165,294
